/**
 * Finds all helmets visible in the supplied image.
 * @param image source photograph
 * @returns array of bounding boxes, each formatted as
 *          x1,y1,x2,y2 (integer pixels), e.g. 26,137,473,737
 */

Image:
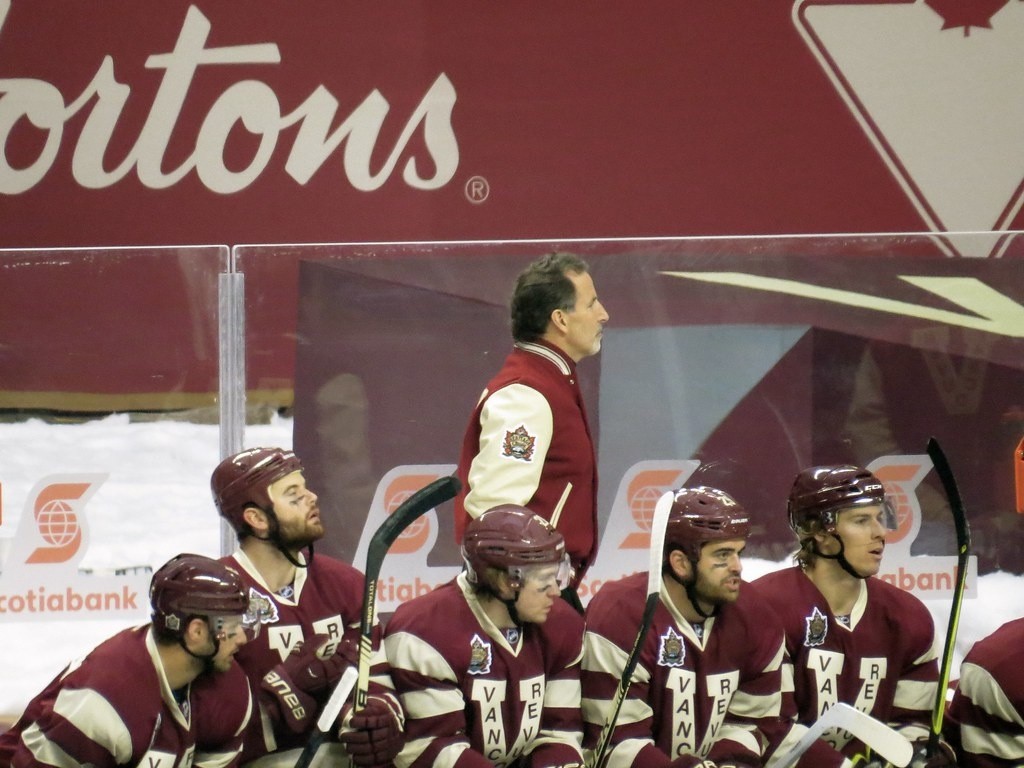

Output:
789,464,895,538
464,504,573,591
211,446,305,514
664,486,751,561
149,554,250,644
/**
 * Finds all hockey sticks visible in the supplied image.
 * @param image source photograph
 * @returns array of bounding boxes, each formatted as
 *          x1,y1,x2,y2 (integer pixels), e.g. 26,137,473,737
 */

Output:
586,490,678,767
772,703,915,768
923,435,972,759
349,475,464,768
293,664,360,768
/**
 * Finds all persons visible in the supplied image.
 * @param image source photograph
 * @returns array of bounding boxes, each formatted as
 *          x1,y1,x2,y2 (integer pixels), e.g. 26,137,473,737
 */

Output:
382,504,588,768
210,446,407,768
747,463,957,768
581,486,799,768
453,252,610,617
942,616,1024,768
0,553,254,768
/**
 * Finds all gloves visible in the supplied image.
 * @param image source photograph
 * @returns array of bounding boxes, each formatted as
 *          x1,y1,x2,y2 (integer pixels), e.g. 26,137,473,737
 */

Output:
291,640,358,694
342,690,405,766
